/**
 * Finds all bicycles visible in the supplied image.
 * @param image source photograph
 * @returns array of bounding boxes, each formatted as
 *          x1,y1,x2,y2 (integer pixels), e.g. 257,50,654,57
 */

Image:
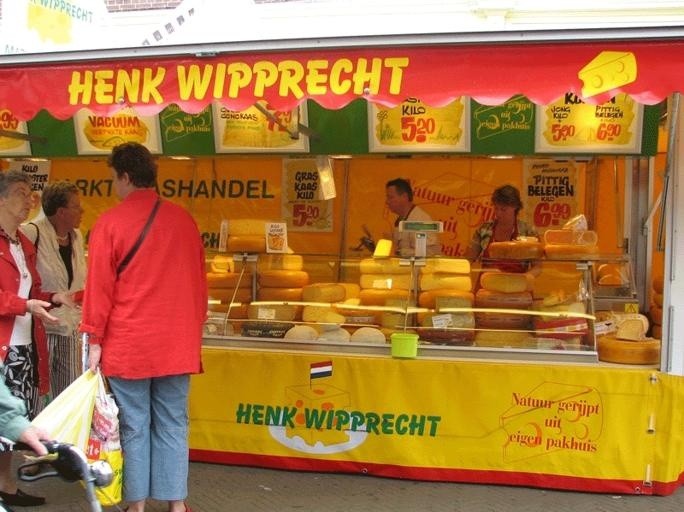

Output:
1,438,116,512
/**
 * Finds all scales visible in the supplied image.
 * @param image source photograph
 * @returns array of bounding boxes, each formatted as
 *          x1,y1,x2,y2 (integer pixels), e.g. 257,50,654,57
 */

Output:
398,220,444,257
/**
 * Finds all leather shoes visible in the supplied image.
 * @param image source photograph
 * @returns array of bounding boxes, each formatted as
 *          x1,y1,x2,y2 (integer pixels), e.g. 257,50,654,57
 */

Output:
0,488,43,506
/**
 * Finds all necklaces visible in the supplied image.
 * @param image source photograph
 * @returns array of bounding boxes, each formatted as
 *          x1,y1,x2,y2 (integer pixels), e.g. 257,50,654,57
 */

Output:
57,231,69,242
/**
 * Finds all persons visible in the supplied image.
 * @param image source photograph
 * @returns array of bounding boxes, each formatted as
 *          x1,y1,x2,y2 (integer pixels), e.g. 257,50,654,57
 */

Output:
19,179,88,416
0,169,78,420
384,177,443,259
0,372,50,456
464,184,543,300
78,141,210,512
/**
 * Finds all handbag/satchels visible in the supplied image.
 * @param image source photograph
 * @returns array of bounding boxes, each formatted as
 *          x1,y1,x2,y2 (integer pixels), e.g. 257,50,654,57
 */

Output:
80,371,123,507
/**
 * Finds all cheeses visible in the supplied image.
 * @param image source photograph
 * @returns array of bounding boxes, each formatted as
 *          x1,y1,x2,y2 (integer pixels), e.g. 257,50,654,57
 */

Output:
207,220,660,366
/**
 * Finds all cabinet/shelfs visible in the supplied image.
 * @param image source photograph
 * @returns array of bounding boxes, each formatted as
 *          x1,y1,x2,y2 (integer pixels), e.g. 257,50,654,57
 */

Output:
203,251,659,365
